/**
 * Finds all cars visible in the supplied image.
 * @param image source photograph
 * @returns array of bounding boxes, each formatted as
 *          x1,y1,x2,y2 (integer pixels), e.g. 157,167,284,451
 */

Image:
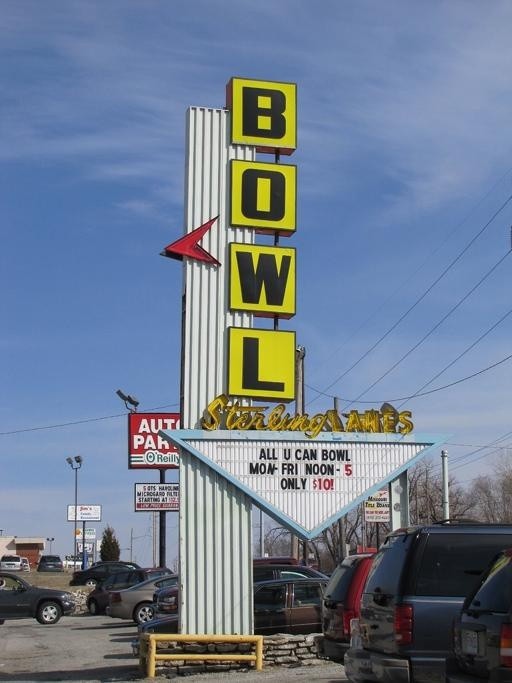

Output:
69,560,332,656
0,572,76,624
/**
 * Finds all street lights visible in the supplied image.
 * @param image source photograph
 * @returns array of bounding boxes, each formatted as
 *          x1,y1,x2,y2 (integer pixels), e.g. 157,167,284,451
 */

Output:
116,388,138,413
48,537,55,554
67,454,82,571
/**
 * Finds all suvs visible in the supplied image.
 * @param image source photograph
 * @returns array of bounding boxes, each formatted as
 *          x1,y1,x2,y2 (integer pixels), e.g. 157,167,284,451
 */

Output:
315,516,512,683
1,555,64,572
